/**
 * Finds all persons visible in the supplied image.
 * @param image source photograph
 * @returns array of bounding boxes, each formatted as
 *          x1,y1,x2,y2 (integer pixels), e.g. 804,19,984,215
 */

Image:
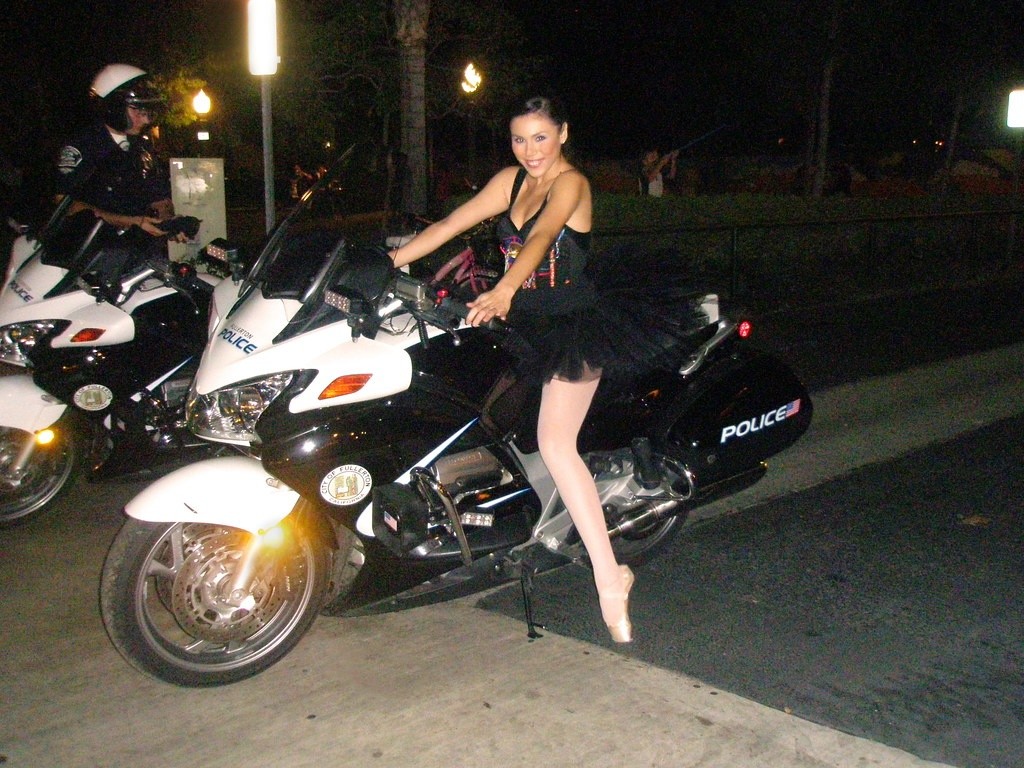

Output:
387,83,636,644
45,60,191,275
637,147,686,198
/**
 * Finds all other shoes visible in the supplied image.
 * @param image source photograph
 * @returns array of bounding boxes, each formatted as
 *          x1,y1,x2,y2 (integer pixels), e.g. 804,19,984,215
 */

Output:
599,564,634,642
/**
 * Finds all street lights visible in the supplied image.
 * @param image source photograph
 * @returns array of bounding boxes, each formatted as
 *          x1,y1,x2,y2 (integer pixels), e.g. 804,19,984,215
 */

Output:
460,60,482,189
192,87,214,159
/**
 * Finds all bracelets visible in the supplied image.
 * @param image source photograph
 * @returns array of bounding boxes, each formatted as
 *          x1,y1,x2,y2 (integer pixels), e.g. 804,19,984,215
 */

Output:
128,216,132,228
140,215,144,228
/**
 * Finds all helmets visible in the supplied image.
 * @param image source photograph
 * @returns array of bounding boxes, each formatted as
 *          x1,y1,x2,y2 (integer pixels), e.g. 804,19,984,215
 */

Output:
89,64,162,132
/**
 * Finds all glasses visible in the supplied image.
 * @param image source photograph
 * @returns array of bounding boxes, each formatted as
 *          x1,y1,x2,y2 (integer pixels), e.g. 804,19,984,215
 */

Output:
127,105,159,117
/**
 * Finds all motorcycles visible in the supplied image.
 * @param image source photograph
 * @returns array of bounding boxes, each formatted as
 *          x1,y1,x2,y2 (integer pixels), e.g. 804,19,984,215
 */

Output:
99,141,812,688
1,152,235,526
